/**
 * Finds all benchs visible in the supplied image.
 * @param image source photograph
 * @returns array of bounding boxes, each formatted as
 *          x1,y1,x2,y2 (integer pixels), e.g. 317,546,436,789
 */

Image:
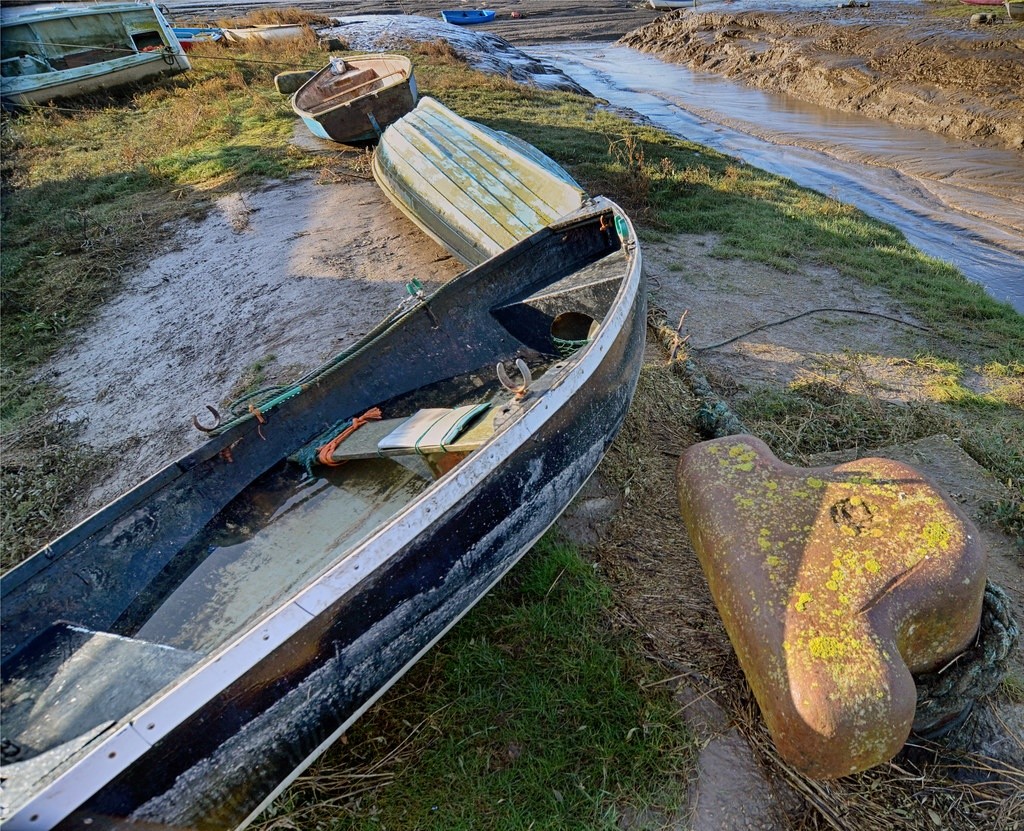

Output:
302,68,403,111
308,404,524,461
0,621,202,751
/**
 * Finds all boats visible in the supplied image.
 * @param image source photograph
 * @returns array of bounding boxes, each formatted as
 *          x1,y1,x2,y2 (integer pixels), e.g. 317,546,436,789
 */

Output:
441,9,495,24
0,0,191,115
372,95,590,267
0,182,647,831
171,27,225,52
291,52,418,144
